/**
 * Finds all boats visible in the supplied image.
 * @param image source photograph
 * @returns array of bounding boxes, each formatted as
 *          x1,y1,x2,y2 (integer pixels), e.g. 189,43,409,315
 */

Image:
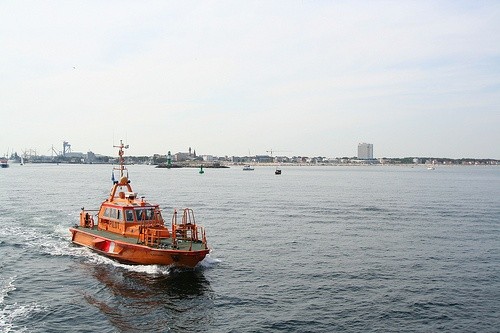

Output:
427,167,435,170
243,165,254,171
67,140,210,269
274,169,281,175
